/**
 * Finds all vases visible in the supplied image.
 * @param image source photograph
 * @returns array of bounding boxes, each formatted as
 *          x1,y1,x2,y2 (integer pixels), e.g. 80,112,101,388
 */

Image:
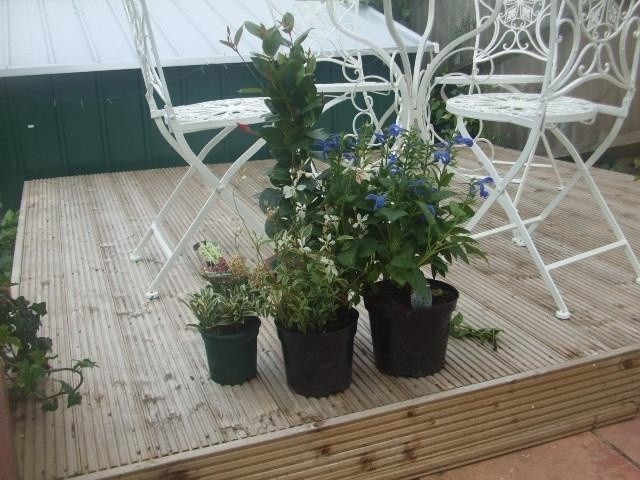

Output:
196,278,458,398
